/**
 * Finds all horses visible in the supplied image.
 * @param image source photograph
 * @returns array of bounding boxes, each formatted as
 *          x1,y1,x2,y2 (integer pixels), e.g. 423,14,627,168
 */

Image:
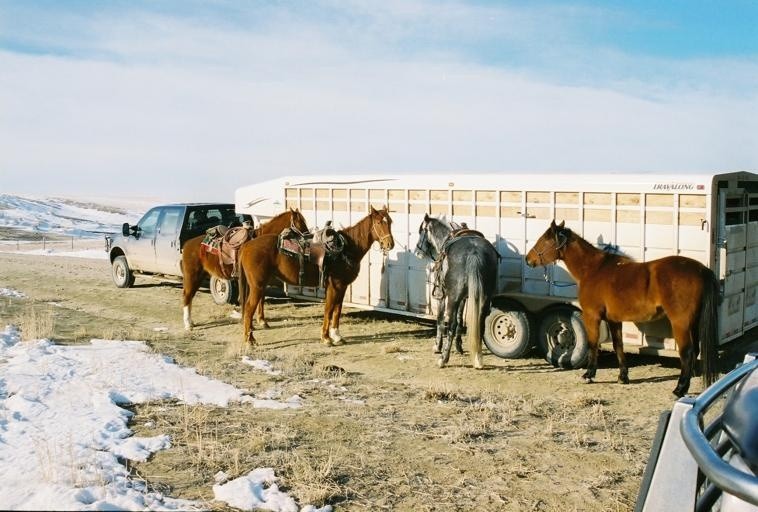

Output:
234,203,396,350
523,218,719,402
178,207,310,333
412,212,499,370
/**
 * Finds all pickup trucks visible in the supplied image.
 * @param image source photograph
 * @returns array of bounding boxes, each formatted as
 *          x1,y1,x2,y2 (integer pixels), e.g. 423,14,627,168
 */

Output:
105,203,254,305
